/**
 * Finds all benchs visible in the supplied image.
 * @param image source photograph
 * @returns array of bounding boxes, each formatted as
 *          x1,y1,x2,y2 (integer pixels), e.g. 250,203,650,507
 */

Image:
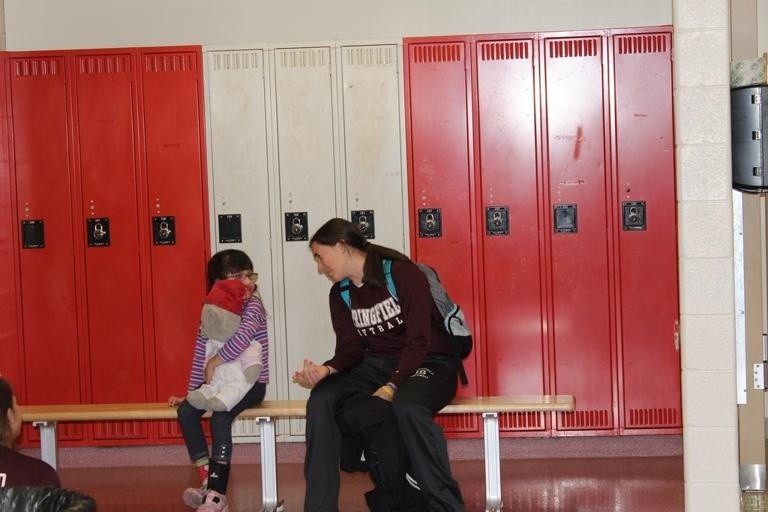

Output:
18,394,576,512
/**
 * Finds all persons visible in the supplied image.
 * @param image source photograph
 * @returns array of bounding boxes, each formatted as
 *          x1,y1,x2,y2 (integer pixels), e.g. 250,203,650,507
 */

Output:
0,375,61,489
288,217,468,512
167,248,271,512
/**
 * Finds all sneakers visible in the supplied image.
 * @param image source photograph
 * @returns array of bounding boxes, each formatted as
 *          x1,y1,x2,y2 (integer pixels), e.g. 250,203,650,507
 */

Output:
196,490,230,512
183,488,206,508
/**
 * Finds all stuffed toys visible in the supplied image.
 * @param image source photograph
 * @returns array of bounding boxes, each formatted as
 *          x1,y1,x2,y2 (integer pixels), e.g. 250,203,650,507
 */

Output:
185,278,264,412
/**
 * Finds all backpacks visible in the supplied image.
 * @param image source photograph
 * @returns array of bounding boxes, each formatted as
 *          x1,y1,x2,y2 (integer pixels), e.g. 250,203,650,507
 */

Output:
340,258,473,359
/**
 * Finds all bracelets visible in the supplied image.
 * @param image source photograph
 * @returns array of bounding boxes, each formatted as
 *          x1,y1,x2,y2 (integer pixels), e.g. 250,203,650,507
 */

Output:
385,380,400,391
380,383,396,399
323,364,331,375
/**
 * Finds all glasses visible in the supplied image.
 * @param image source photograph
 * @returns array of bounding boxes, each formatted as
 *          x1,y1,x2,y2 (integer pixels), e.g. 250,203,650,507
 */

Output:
224,273,258,283
